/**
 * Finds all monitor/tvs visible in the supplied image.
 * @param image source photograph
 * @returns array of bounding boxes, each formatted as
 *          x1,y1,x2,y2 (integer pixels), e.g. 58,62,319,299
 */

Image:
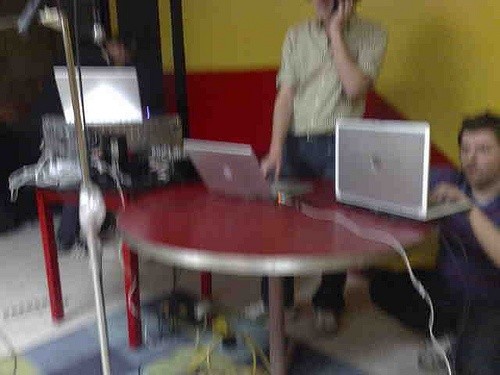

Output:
53,65,146,126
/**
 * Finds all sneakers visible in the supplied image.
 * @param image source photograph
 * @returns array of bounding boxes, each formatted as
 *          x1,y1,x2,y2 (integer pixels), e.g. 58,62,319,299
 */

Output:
311,304,338,335
239,301,296,324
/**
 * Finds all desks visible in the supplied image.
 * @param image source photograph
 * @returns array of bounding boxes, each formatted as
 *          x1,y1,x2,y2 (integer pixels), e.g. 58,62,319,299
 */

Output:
116,173,432,375
34,178,213,327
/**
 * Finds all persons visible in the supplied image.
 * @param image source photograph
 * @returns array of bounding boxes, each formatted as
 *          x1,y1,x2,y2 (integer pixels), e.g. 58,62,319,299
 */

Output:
241,0,389,333
370,110,500,375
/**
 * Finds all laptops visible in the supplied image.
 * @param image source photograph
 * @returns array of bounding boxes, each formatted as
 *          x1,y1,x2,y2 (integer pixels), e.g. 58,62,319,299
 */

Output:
335,116,452,222
183,139,311,202
